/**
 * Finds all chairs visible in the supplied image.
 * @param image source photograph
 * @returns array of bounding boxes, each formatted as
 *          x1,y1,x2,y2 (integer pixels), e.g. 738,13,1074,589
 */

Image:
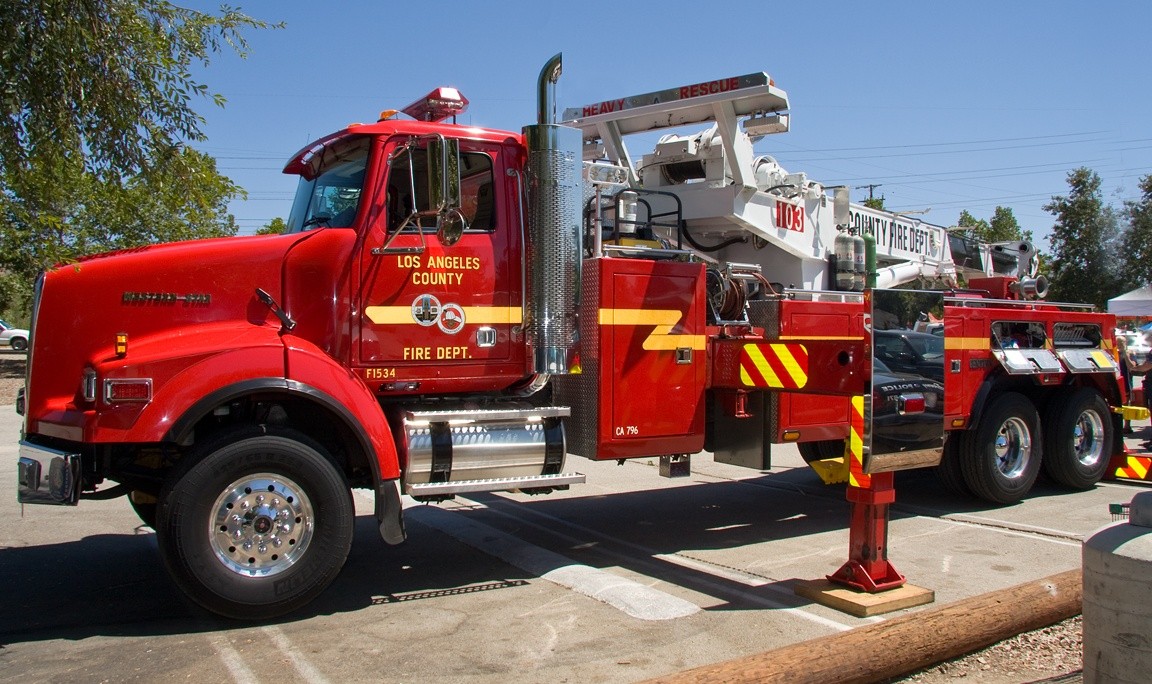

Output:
470,183,495,230
875,344,893,362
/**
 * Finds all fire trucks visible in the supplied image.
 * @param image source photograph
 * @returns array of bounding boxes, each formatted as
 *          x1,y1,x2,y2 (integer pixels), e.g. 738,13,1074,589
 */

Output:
15,48,1152,626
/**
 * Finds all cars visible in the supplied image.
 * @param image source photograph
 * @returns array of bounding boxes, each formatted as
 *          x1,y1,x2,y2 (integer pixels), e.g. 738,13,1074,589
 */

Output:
0,319,30,351
